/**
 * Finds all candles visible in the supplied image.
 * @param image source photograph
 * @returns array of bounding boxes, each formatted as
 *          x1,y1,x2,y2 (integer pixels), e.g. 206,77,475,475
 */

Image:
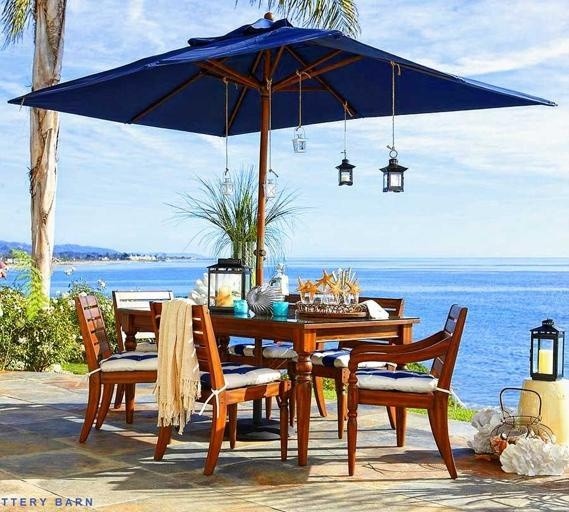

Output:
390,174,397,186
539,349,553,374
342,175,349,181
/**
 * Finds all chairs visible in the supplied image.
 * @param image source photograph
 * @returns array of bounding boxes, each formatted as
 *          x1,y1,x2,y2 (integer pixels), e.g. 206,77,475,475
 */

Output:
72,281,469,481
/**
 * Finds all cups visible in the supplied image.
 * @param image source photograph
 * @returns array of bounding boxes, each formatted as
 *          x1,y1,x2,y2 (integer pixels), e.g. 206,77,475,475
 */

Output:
272,302,289,322
233,299,249,315
300,290,360,305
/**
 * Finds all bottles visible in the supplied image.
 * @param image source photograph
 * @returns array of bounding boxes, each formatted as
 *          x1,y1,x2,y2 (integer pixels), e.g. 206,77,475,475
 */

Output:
275,265,289,296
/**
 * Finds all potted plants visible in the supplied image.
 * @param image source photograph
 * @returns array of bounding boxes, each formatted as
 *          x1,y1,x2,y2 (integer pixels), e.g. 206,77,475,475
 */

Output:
162,162,315,289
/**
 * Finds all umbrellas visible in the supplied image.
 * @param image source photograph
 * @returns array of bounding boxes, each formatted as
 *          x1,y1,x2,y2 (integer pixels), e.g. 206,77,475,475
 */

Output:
6,12,558,440
